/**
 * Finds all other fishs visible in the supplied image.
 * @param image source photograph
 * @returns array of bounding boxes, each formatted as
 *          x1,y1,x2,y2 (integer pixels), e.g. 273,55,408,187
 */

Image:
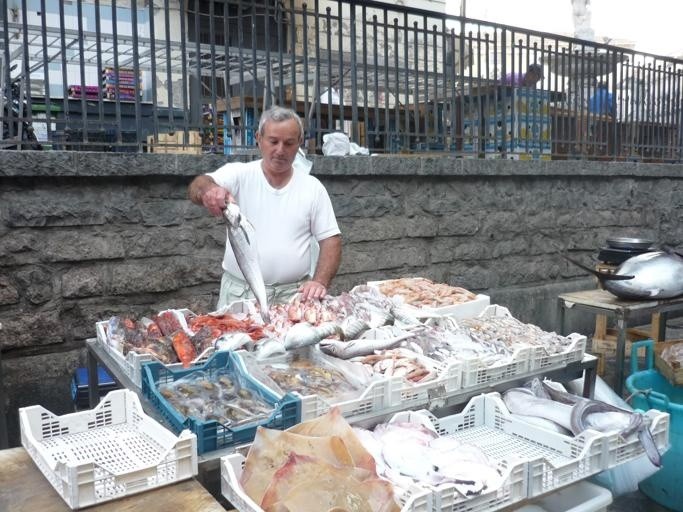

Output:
223,201,271,323
104,276,663,512
566,248,683,299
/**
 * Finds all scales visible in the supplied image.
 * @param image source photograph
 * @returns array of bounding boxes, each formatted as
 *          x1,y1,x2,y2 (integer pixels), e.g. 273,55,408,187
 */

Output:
70,368,119,407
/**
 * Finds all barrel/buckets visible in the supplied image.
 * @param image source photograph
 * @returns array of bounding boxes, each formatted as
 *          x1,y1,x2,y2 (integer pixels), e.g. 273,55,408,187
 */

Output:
624,338,683,512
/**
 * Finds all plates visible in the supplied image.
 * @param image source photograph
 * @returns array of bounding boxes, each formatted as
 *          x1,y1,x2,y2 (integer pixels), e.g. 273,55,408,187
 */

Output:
602,237,655,250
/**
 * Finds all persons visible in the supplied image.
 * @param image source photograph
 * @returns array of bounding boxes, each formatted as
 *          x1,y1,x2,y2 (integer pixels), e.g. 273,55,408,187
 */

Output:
497,62,543,88
589,81,613,146
186,104,342,314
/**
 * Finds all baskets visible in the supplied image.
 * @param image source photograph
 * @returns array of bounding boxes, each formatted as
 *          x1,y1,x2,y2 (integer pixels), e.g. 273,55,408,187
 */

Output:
224,380,669,512
96,277,587,457
19,388,199,510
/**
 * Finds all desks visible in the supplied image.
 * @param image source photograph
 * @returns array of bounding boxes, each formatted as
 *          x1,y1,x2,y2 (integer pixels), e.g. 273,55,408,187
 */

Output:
556,290,683,396
1,439,228,512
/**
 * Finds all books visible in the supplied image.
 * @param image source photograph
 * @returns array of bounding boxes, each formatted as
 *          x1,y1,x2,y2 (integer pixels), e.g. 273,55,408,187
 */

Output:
66,67,146,99
